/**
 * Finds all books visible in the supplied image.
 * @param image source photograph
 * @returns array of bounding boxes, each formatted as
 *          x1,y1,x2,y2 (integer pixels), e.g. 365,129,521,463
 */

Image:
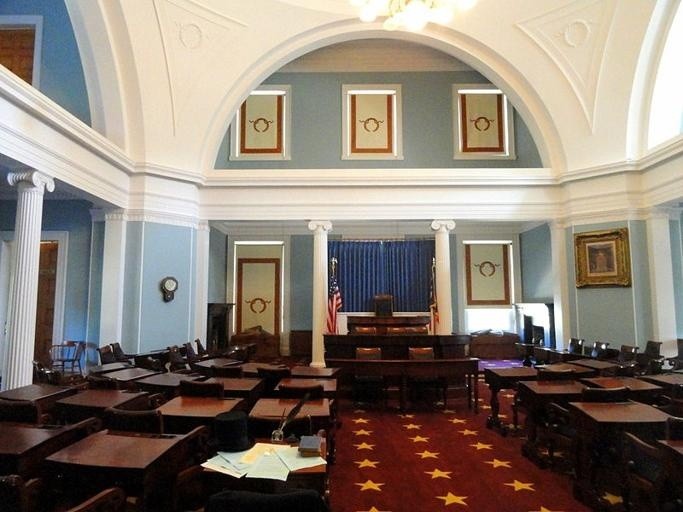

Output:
298,434,321,457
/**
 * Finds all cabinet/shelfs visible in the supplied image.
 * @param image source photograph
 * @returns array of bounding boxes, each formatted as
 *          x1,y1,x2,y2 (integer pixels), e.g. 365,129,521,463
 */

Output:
208,302,236,350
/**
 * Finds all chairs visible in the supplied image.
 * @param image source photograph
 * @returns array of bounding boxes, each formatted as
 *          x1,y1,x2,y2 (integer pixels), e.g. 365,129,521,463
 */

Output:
405,345,440,405
482,337,683,512
350,323,429,336
353,345,387,403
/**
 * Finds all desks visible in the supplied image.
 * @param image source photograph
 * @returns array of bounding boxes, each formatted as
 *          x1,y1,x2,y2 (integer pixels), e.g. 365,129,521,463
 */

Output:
322,355,480,415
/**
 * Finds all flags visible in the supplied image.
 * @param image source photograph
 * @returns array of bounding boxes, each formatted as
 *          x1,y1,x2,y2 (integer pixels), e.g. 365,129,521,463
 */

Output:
326,268,343,332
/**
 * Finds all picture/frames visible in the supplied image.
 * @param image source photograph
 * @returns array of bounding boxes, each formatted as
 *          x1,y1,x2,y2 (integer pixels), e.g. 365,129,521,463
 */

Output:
574,227,630,289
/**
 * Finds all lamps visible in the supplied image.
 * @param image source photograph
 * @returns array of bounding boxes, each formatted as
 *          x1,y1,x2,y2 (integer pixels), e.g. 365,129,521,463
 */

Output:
348,1,478,35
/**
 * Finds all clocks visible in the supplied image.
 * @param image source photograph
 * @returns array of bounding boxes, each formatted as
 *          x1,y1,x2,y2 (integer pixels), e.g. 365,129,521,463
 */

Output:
161,276,178,302
